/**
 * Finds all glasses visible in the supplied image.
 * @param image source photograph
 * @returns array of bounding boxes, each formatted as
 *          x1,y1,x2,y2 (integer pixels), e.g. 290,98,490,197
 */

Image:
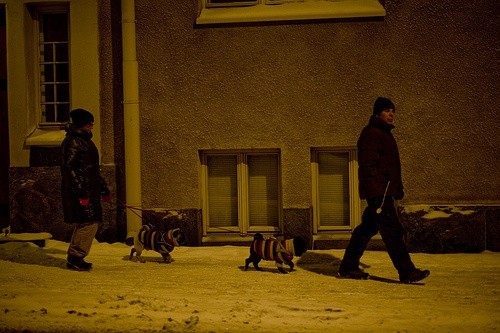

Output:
87,123,94,128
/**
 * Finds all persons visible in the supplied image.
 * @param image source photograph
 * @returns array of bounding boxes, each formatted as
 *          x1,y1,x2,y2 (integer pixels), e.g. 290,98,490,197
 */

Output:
338,96,430,284
59,109,112,270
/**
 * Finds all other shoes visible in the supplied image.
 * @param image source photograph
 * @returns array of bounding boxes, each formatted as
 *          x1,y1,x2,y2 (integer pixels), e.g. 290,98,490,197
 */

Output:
337,270,370,280
399,268,431,284
66,257,93,271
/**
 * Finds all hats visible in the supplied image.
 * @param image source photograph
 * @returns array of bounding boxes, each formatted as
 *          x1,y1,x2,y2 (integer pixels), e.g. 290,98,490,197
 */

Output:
373,96,396,116
69,108,95,129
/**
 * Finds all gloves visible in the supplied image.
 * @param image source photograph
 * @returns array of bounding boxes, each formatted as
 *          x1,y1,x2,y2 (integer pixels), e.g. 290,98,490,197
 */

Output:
79,198,91,207
102,193,112,203
393,189,405,200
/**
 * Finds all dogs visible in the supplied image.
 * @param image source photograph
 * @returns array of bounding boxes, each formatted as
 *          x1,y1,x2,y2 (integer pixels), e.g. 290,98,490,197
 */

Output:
243,233,307,274
126,225,186,264
